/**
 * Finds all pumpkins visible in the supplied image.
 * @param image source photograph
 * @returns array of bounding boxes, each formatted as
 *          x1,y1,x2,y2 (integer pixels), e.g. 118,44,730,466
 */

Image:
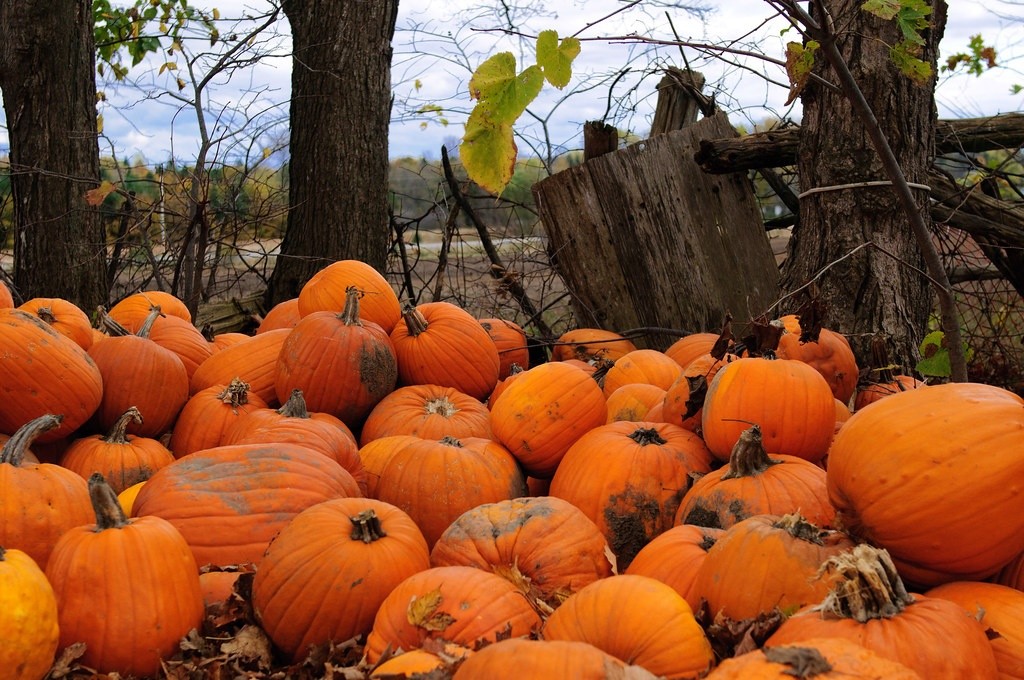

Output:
0,260,1024,680
